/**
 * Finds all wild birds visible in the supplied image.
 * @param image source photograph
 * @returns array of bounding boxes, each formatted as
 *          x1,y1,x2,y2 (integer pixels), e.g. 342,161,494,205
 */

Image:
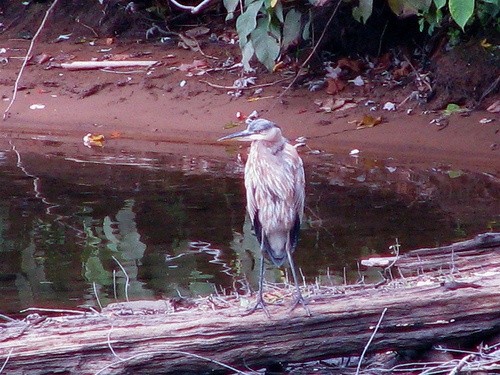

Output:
217,117,313,318
